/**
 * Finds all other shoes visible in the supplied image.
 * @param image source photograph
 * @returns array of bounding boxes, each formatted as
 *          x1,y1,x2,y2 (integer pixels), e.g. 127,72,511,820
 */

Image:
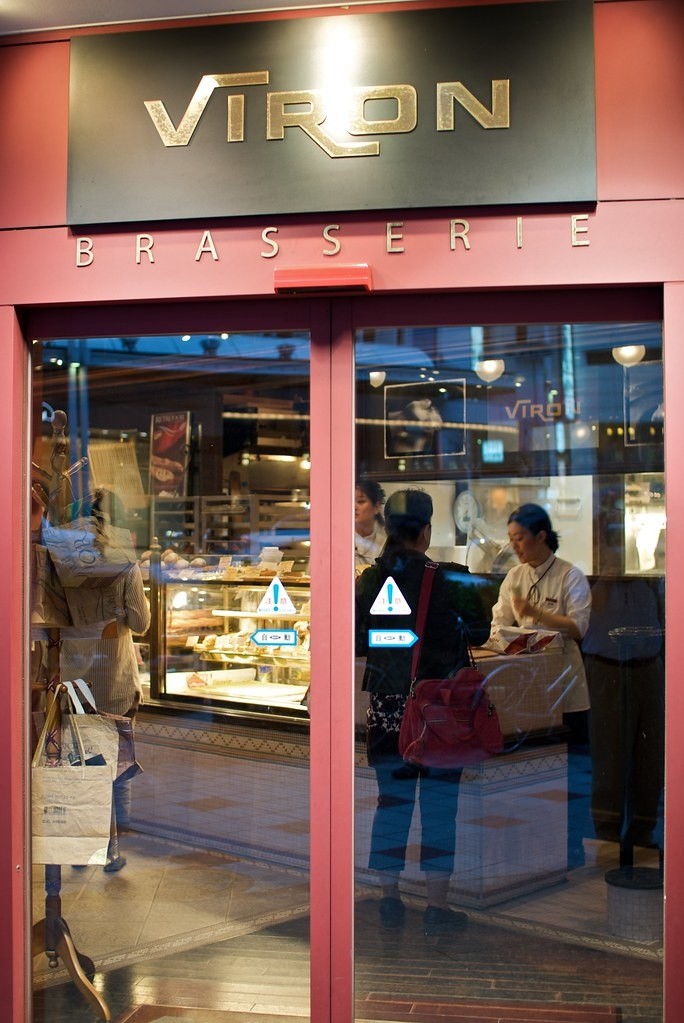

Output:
423,905,468,934
379,897,406,928
567,845,586,867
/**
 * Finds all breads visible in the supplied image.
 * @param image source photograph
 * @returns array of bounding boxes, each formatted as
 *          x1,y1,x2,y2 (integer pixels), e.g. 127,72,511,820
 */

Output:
137,549,207,570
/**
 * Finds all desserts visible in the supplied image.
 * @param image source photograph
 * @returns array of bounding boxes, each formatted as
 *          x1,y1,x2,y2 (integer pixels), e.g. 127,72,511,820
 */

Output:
186,605,310,689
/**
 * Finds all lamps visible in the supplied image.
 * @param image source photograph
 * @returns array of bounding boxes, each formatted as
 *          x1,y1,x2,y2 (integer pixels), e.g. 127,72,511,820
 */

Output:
474,358,505,382
612,345,646,369
370,371,387,388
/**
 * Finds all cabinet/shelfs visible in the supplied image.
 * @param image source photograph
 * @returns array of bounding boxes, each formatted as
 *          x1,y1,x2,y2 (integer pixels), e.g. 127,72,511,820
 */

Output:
131,554,312,725
123,496,310,559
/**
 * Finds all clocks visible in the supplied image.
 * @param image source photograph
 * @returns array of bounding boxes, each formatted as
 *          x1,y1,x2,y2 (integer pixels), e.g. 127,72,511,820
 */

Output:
452,491,482,533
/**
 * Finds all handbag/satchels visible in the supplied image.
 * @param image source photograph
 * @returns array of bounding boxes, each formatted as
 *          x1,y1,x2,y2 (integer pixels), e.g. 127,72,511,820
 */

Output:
30,679,146,866
479,624,565,656
398,560,504,769
30,471,137,635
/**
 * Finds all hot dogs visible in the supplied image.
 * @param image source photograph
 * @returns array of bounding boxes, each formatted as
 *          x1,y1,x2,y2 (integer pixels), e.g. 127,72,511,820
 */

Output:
150,455,182,495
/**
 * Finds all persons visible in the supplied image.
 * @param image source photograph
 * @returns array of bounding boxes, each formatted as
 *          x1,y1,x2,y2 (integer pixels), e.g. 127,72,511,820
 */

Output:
487,502,592,869
355,491,463,935
60,491,147,871
350,477,383,570
